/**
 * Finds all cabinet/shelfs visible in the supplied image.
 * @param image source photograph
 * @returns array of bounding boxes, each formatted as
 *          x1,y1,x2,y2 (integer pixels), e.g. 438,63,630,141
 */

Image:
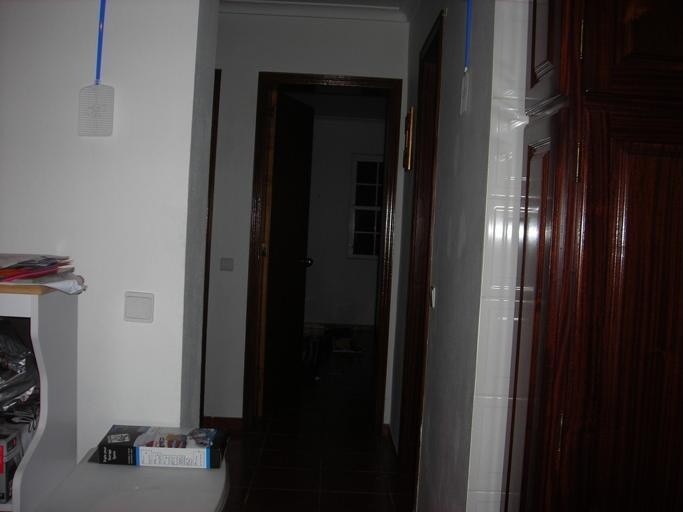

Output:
4,277,228,511
